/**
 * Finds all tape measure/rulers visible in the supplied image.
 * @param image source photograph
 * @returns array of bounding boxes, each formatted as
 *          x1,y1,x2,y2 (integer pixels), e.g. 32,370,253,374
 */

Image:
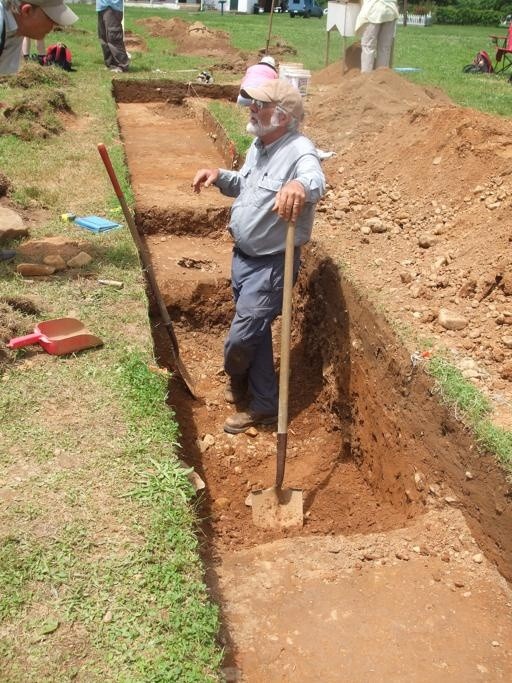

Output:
58,213,76,222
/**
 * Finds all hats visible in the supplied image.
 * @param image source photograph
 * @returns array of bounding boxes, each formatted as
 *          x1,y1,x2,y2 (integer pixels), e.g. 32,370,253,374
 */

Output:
259,55,278,73
34,0,79,27
239,79,304,119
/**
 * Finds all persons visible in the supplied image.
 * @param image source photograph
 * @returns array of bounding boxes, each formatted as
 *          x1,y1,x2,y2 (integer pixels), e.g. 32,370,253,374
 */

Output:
22,36,46,63
96,0,130,72
0,0,80,73
236,56,276,107
191,81,326,434
354,0,400,71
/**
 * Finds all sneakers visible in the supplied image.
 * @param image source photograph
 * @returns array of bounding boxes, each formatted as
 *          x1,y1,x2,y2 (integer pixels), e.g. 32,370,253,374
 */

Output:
224,360,248,403
223,408,278,434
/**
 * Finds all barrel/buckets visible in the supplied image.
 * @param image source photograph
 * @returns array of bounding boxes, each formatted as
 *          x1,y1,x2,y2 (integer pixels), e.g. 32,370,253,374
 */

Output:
284,68,312,97
278,61,304,79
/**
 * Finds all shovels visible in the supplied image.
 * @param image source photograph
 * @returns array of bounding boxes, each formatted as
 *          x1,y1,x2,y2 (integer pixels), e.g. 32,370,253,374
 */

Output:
5,317,104,356
251,219,303,531
96,142,197,401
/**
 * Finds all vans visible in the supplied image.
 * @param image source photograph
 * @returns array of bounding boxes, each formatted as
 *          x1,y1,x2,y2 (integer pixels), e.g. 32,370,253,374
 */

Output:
288,0,324,19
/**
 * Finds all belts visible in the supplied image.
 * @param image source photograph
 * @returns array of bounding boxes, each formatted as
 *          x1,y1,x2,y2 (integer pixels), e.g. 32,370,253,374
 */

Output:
231,246,252,260
